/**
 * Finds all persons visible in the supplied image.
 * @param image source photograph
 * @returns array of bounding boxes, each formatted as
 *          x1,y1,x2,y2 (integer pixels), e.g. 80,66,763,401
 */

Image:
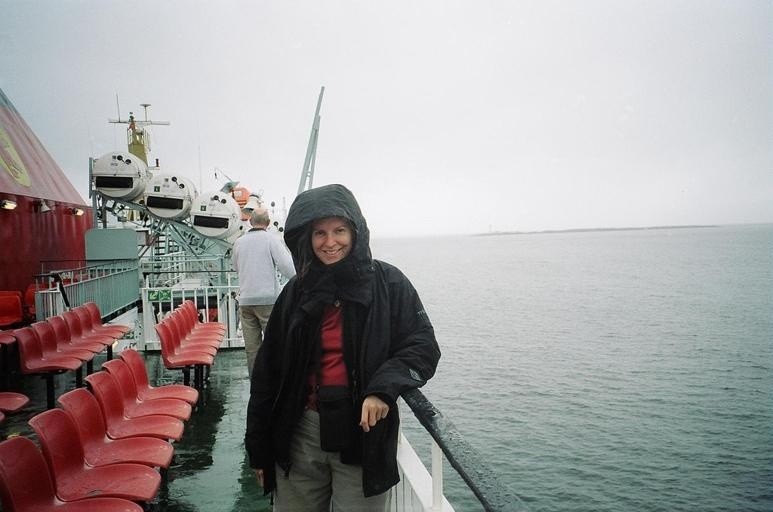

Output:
241,184,443,512
229,206,295,386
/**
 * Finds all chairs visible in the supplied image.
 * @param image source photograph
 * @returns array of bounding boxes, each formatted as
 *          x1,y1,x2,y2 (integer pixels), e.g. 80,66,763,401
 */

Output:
0,281,230,512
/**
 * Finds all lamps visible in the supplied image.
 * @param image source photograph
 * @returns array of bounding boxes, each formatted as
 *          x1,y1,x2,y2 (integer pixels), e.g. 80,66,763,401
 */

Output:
37,197,52,214
1,199,17,211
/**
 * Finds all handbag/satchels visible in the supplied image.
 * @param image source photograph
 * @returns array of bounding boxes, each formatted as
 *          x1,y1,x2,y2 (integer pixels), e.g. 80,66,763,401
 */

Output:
315,386,355,453
